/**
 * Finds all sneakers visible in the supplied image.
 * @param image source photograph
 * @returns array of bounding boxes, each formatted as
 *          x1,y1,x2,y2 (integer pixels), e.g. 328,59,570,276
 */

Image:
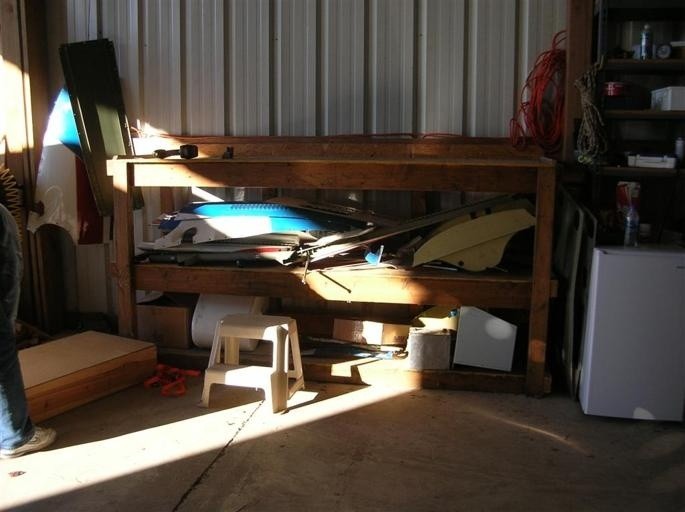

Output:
0,425,57,458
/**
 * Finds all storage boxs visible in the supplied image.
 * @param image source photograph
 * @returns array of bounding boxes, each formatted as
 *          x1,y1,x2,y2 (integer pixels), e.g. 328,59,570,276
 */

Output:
15,330,157,424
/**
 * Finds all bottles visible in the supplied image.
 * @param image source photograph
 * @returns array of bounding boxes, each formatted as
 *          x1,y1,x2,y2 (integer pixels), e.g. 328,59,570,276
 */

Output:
448,309,459,334
639,24,652,62
623,206,639,247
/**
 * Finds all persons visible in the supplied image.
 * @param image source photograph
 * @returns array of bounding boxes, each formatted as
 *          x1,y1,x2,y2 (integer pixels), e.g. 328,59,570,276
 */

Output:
0,203,58,459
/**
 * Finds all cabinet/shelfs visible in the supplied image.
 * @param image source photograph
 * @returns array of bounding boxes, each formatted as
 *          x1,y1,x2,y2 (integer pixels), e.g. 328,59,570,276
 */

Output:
561,0,685,254
106,135,557,400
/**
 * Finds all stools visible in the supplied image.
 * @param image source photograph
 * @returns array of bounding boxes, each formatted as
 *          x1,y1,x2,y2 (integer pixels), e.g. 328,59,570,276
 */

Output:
198,312,305,414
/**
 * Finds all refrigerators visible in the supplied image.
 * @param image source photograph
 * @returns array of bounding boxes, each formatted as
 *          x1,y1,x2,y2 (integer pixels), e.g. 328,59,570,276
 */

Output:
580,248,684,423
